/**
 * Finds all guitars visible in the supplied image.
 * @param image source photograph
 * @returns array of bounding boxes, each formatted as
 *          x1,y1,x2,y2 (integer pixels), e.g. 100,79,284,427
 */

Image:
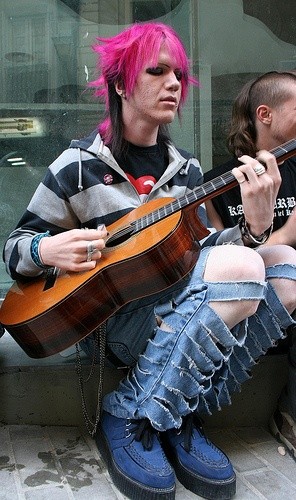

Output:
0,137,296,359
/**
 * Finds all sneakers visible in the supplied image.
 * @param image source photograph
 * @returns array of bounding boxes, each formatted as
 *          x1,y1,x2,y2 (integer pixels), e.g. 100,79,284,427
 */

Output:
93,412,177,500
160,413,238,500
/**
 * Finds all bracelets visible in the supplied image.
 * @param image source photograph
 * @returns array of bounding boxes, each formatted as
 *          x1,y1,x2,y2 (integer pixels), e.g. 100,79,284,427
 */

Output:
242,216,268,245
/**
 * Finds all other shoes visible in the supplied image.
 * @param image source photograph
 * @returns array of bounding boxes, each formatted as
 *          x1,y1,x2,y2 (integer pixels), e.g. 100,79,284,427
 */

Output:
269,408,296,459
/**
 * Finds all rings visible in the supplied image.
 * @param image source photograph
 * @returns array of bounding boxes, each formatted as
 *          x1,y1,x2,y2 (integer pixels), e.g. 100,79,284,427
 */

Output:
86,240,93,252
254,164,265,176
87,249,99,262
238,179,247,184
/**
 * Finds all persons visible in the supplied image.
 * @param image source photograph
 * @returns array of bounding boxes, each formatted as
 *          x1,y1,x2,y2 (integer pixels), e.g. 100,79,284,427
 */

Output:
203,70,296,461
2,22,296,500
